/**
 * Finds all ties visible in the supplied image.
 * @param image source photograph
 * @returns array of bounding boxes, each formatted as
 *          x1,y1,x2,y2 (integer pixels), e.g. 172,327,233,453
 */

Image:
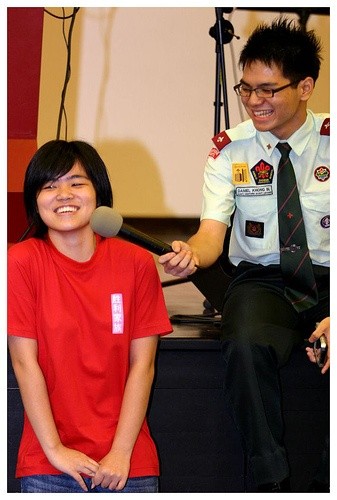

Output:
276,142,319,312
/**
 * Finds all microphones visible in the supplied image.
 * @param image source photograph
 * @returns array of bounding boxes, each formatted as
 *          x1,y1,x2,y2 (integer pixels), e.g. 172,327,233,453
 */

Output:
91,206,173,258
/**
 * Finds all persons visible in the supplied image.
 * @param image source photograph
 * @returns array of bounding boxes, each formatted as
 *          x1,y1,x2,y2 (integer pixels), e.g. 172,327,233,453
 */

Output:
7,139,173,493
159,14,330,492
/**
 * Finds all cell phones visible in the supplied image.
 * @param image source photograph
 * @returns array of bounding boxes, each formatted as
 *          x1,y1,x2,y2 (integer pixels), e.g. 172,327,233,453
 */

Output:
313,322,327,368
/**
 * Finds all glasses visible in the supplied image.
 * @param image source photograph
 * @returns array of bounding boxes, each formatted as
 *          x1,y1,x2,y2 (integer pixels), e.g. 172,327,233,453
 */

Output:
233,80,299,98
